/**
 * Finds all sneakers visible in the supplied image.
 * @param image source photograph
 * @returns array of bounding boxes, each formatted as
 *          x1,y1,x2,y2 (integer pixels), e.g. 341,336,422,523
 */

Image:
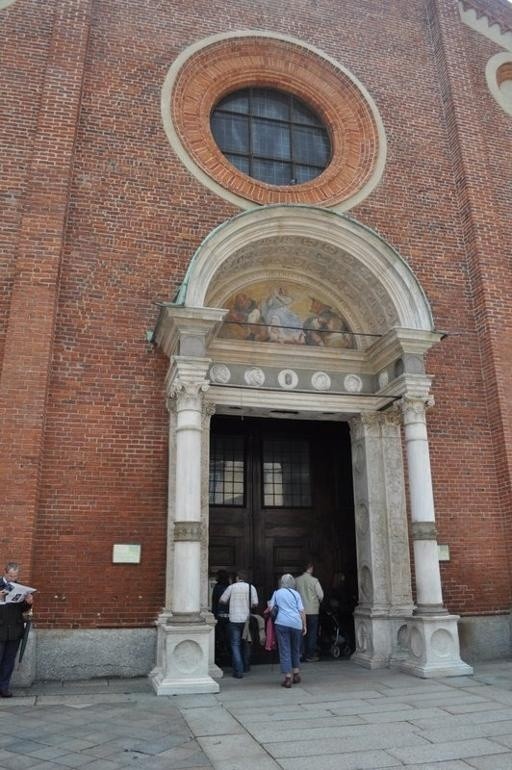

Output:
243,663,251,674
304,655,320,663
232,670,244,679
291,673,302,684
280,677,292,688
0,687,13,698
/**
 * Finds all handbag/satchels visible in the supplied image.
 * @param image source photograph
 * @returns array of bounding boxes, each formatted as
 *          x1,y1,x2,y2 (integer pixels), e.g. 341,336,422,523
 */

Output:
248,614,266,644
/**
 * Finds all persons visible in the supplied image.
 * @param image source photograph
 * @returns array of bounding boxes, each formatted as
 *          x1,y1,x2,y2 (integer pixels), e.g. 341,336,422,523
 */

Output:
263,573,308,688
217,568,259,678
211,568,231,666
294,561,326,663
0,561,35,698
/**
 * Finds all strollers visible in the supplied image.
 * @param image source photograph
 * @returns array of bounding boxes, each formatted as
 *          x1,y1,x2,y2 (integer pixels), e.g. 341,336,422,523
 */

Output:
315,609,354,658
